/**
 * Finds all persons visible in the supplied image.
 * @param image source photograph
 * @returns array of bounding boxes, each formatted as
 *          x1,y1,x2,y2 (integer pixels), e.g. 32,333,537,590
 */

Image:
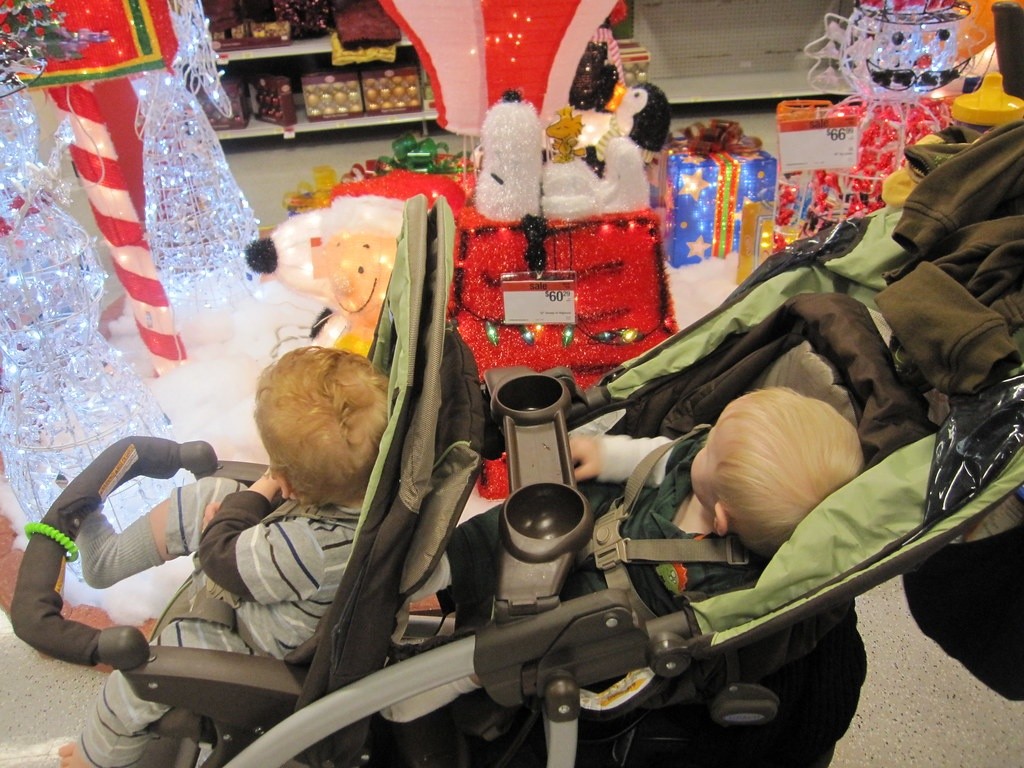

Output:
58,346,389,768
378,387,865,722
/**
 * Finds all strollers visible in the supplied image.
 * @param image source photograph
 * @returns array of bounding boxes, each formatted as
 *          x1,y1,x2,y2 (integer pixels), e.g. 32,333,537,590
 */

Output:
11,195,1024,768
